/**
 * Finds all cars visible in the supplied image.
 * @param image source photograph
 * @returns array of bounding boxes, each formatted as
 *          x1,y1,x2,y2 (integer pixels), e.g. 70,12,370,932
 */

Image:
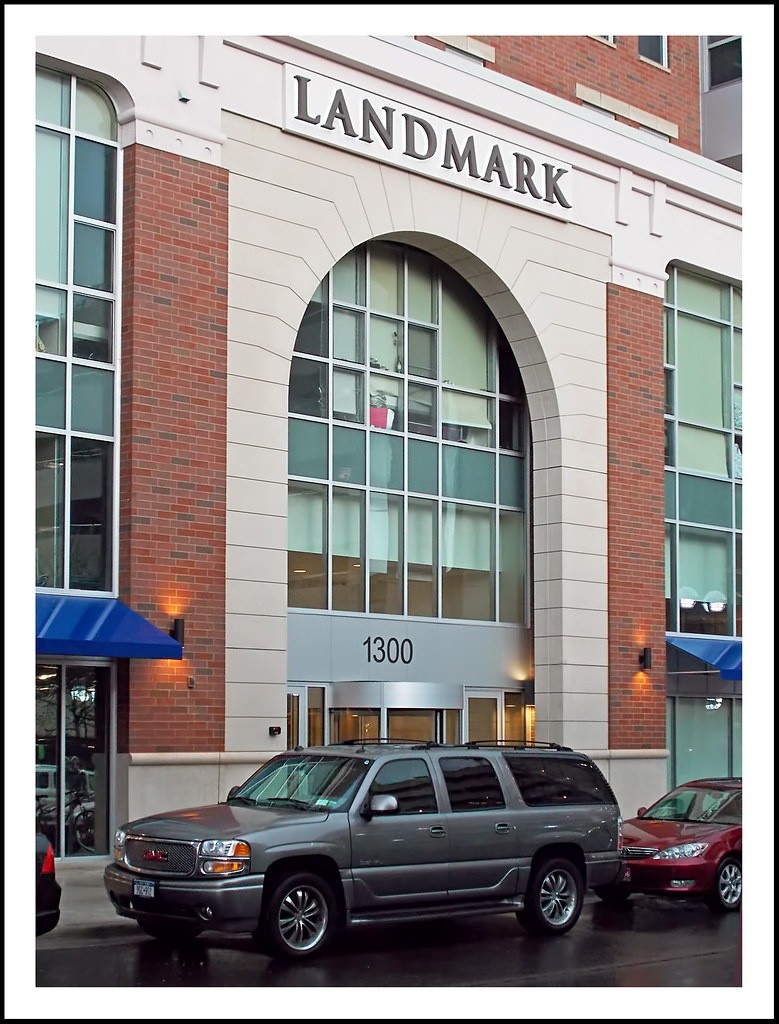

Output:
599,777,743,914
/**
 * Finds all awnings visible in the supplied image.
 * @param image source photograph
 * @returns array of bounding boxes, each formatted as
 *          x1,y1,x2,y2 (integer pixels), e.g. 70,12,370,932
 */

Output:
664,633,742,682
36,594,184,659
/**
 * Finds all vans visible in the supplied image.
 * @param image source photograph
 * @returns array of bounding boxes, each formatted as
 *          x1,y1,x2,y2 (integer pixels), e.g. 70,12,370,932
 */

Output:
35,764,96,825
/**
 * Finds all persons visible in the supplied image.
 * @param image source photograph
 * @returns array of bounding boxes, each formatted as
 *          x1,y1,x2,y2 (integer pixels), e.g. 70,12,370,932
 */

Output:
55,753,95,797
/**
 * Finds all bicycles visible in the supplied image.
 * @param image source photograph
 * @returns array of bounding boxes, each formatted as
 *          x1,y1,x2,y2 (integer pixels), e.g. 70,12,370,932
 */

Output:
36,781,96,854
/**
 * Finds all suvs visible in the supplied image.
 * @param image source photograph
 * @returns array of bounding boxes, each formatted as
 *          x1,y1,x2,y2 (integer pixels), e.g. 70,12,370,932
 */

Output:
102,737,627,961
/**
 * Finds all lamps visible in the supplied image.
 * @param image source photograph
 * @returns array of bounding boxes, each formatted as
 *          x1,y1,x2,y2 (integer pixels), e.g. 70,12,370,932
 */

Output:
639,648,652,670
170,619,185,648
521,680,534,706
